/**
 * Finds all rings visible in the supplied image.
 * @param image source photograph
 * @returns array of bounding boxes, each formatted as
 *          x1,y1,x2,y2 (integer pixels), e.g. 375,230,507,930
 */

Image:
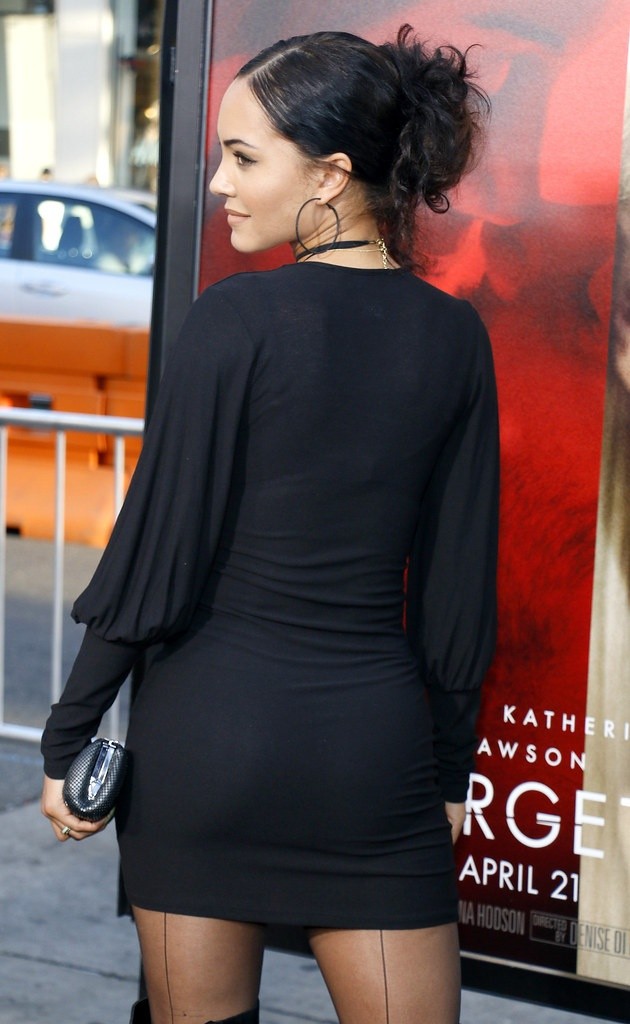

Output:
62,827,71,836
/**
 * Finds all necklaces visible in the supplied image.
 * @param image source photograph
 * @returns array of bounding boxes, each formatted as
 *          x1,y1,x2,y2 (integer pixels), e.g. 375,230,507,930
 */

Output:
297,238,389,270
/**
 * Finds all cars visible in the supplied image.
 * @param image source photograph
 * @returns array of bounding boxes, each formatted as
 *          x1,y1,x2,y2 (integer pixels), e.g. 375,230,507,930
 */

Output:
0,183,156,327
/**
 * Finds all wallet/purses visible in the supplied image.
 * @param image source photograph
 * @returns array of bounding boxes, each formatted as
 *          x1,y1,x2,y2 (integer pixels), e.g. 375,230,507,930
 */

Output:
61,738,127,823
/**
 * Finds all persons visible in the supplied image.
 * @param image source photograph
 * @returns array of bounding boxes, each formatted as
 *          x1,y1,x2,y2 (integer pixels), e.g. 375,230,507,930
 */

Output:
41,30,499,1024
90,219,149,275
